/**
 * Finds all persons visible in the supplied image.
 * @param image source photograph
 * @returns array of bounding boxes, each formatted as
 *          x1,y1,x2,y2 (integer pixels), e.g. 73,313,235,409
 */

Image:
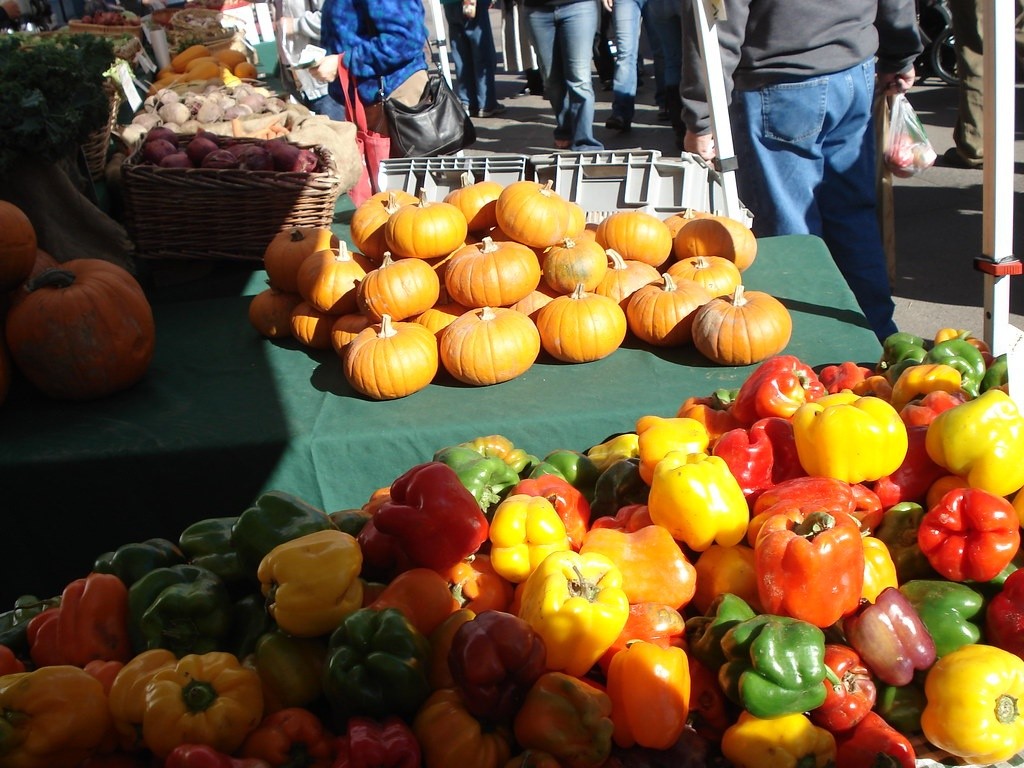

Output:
680,0,925,348
271,0,687,158
0,0,169,34
943,0,1024,170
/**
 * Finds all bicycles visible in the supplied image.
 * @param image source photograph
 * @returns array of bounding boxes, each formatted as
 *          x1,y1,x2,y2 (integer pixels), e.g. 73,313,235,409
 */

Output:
934,27,961,85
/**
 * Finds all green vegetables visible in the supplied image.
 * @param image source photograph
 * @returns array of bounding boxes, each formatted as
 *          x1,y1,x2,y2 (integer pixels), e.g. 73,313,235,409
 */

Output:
0,32,132,154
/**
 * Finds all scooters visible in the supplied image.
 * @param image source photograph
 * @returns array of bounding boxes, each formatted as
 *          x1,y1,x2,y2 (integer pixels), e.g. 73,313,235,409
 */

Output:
914,0,954,82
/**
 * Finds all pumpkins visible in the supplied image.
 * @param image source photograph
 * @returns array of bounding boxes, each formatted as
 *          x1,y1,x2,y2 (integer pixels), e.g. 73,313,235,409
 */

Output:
251,171,792,400
0,200,153,396
150,45,258,94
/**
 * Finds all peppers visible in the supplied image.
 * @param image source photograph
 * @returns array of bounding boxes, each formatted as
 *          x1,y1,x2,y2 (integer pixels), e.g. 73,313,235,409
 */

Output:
885,134,937,177
0,328,1024,768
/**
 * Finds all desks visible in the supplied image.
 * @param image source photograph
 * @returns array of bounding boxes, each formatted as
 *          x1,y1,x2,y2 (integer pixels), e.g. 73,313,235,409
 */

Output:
0,230,887,617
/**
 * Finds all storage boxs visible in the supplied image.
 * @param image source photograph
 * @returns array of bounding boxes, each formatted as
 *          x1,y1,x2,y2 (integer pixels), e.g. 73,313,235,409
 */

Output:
380,146,756,231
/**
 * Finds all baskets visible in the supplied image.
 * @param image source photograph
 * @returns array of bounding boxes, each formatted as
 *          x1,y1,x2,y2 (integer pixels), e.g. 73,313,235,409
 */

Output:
16,10,337,263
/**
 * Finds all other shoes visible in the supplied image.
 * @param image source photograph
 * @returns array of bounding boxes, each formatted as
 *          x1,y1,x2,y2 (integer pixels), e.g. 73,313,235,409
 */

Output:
517,68,542,96
657,107,671,121
944,146,983,169
606,112,632,131
553,126,572,149
460,102,506,118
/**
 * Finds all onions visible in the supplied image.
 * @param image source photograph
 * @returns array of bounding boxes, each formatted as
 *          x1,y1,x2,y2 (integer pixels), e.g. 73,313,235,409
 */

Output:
120,87,318,173
82,13,142,26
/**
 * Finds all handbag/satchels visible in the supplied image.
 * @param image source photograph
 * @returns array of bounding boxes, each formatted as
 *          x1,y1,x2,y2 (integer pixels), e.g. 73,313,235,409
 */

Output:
385,74,476,161
884,82,938,179
337,50,391,210
